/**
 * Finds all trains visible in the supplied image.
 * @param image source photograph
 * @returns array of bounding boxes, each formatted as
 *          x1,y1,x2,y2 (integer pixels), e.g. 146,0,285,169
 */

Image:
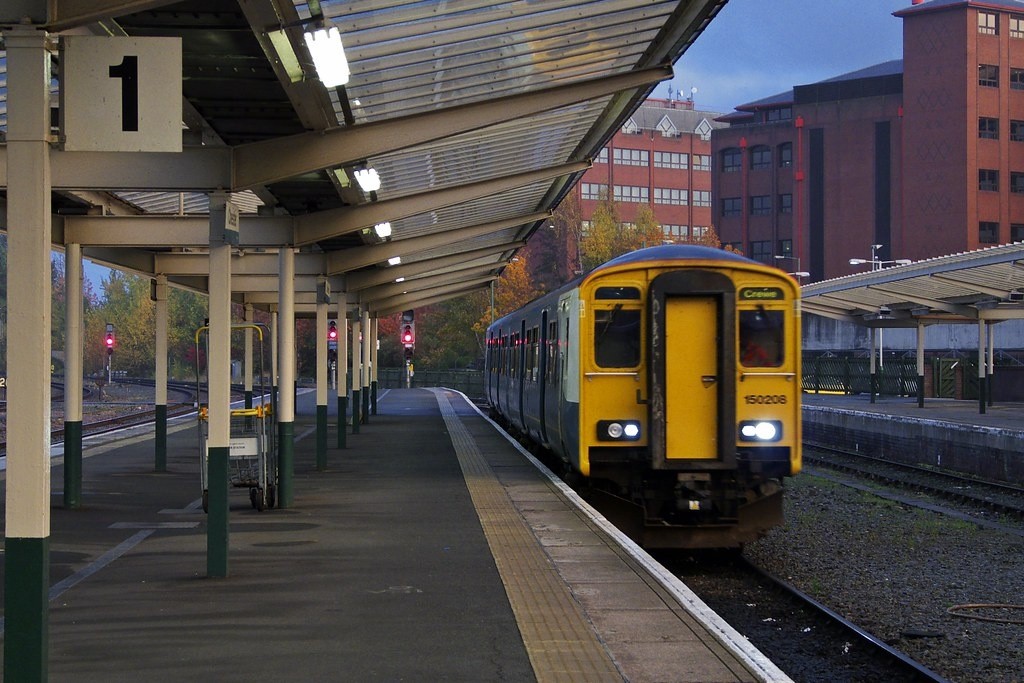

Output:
485,241,801,557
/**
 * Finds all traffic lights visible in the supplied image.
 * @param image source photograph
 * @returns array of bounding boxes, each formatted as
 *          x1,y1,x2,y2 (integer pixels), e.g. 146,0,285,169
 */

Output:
105,323,114,356
401,310,414,343
328,320,337,340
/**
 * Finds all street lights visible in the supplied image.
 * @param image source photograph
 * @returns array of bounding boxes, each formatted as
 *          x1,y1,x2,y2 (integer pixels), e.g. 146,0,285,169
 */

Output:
848,259,912,399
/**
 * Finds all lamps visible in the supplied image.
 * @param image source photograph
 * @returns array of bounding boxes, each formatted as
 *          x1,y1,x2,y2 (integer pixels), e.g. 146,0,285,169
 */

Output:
354,164,381,192
375,222,392,238
303,25,352,90
388,256,401,265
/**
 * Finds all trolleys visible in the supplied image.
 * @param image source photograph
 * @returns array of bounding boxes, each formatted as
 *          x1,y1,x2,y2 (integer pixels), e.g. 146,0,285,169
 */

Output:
195,321,277,511
